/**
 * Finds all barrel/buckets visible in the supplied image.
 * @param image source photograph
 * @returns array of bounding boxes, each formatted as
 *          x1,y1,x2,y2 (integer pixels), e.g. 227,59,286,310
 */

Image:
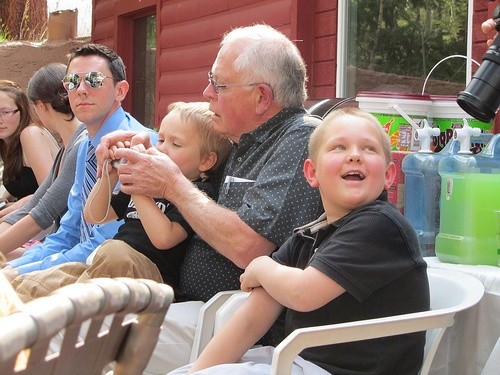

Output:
401,153,444,257
355,90,433,151
429,94,495,153
435,155,500,266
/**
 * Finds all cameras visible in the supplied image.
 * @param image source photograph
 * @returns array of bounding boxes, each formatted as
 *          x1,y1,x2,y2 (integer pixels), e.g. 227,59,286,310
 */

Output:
456,6,500,123
111,157,128,168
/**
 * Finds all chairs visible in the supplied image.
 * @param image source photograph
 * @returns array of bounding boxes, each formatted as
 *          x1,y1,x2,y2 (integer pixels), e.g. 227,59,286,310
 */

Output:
190,267,485,375
0,277,175,375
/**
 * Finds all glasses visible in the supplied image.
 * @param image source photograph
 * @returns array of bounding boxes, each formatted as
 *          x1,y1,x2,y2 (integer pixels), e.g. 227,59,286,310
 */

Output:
0,108,19,116
61,71,121,92
208,72,275,101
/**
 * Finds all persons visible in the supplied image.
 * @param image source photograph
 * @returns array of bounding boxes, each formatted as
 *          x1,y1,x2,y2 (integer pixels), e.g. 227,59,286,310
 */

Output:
167,106,431,375
93,24,327,375
0,44,161,281
9,99,233,305
0,60,90,266
0,80,63,223
480,18,499,47
